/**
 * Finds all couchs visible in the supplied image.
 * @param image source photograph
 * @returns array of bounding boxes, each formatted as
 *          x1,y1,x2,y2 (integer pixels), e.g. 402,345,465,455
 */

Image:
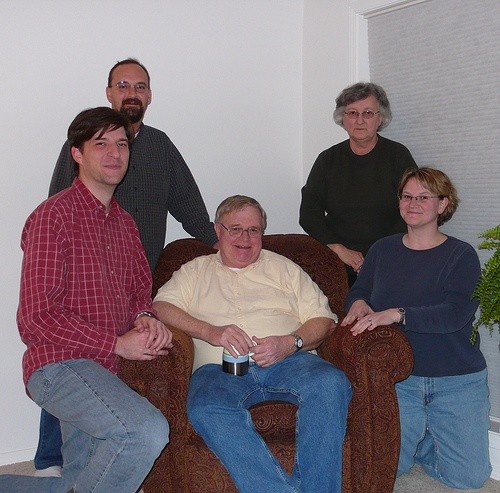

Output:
115,233,413,493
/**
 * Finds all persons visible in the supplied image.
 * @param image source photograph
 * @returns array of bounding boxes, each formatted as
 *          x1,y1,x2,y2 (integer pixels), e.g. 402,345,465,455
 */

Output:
341,167,493,490
33,57,221,477
152,195,351,493
299,83,419,290
0,107,173,493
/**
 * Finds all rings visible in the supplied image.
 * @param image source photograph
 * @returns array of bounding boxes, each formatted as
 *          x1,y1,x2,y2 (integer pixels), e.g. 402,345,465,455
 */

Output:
369,319,372,326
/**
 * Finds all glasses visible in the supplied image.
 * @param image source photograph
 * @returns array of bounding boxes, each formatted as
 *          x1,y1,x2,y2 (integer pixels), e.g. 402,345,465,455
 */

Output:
109,82,149,93
402,195,440,202
343,110,380,119
219,222,263,238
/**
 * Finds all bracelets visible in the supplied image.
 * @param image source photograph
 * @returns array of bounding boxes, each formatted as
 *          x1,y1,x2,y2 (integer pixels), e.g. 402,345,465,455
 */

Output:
135,312,153,320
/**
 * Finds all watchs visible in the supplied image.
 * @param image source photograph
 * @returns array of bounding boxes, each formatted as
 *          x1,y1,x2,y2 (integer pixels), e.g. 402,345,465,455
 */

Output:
289,333,304,353
394,306,405,324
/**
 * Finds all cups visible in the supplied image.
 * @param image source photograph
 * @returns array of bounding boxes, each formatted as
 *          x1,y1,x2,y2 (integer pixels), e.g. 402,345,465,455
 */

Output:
221,340,258,376
